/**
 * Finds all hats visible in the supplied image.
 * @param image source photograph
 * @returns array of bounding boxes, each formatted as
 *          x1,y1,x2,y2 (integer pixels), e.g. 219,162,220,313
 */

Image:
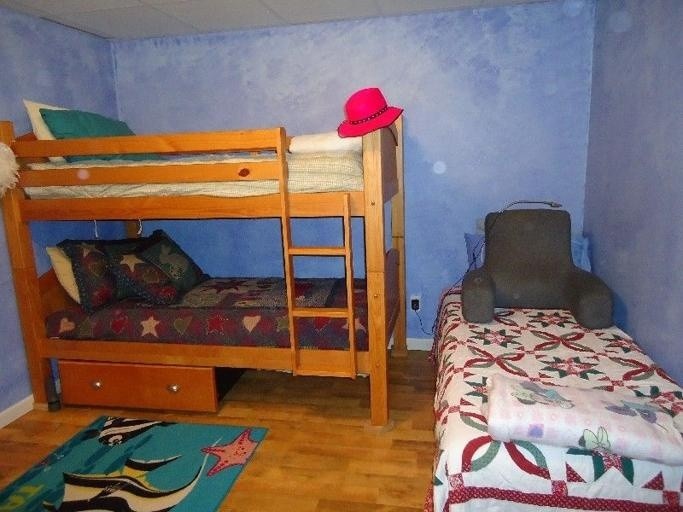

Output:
336,87,406,138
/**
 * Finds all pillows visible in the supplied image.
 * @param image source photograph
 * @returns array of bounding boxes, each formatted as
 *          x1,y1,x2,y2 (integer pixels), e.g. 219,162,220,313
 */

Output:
18,96,71,164
52,233,152,317
40,242,84,308
130,228,212,295
460,229,593,277
97,228,187,308
35,103,166,168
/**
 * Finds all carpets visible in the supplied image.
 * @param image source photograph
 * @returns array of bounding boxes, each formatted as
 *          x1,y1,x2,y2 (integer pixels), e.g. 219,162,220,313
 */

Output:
0,412,272,512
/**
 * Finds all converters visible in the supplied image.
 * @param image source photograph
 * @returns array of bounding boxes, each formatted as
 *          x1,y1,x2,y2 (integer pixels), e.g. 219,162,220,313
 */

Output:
411,299,419,310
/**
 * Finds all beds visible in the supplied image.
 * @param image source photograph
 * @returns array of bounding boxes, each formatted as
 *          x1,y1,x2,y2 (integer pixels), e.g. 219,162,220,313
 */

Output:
0,109,411,428
422,277,682,511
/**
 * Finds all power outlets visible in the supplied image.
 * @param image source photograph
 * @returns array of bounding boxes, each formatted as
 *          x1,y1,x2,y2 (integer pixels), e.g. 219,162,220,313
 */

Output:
408,295,422,314
